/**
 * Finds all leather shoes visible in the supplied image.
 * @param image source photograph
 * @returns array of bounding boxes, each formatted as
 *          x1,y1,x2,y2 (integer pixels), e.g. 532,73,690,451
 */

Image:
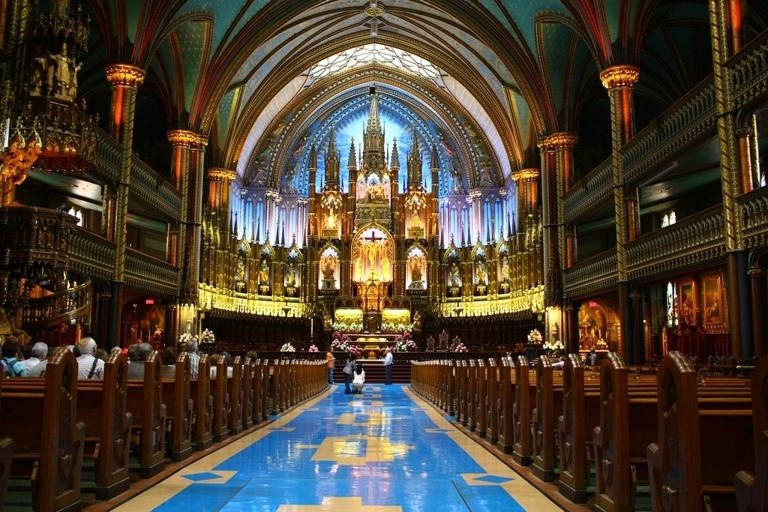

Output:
345,390,361,394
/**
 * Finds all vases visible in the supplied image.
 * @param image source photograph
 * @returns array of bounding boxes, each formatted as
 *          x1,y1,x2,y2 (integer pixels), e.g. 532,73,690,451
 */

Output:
201,342,216,353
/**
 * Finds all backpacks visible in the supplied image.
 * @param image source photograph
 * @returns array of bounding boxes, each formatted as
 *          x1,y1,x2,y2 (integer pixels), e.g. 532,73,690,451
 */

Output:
0,359,17,377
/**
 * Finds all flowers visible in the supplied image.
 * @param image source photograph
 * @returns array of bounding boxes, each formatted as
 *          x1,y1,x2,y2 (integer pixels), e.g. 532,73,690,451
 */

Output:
178,331,195,344
200,328,216,342
279,342,296,353
526,328,543,344
449,343,468,352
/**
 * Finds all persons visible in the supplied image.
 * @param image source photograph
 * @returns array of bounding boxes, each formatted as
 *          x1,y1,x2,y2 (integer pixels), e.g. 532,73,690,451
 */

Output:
586,352,592,360
590,350,597,365
352,364,366,393
0,334,263,381
343,354,357,394
326,346,337,384
46,43,74,97
384,348,395,384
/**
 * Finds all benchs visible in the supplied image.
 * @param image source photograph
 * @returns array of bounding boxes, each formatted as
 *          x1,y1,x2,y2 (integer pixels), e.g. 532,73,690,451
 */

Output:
409,350,767,512
0,348,329,512
